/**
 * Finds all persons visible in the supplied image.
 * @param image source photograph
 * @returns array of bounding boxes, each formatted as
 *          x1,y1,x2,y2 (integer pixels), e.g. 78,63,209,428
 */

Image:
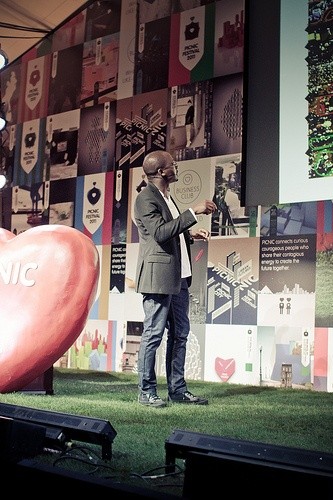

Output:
134,152,219,407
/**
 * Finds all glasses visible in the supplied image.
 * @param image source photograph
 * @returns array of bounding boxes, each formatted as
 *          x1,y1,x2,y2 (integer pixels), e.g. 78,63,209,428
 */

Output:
155,160,177,170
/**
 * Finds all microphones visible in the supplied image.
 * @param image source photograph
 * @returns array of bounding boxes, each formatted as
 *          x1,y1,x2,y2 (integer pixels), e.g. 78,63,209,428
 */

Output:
167,174,177,177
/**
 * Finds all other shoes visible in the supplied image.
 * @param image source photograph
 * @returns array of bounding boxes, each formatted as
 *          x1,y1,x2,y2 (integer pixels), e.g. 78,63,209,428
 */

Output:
137,383,167,408
167,390,208,406
